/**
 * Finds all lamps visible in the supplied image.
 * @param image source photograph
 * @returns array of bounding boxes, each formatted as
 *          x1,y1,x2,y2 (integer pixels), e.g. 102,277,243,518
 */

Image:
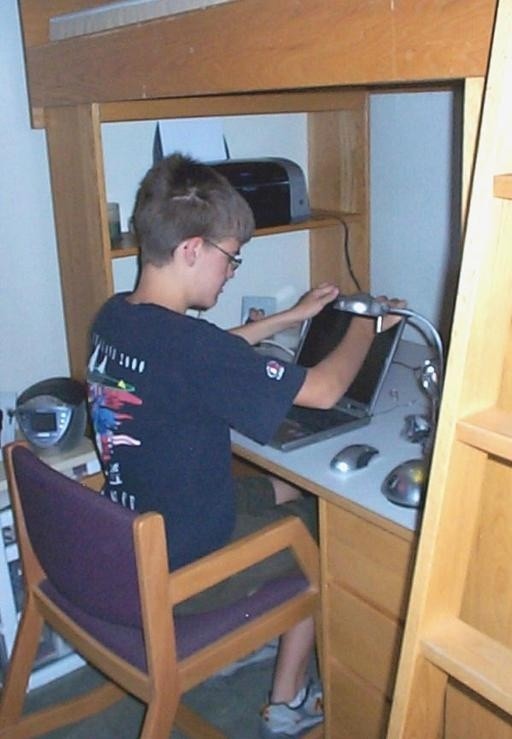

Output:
331,294,445,510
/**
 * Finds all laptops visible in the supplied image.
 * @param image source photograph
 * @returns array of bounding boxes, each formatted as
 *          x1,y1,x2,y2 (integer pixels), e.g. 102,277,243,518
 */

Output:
266,297,406,453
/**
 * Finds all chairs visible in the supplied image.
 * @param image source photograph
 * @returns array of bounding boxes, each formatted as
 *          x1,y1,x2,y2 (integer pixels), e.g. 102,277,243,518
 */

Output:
0,439,320,739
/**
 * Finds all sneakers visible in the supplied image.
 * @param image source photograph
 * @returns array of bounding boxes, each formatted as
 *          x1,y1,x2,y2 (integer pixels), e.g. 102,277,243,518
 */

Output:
262,676,324,737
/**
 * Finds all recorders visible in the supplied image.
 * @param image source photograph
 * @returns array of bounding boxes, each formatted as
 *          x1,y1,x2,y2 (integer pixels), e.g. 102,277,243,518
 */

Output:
14,376,89,457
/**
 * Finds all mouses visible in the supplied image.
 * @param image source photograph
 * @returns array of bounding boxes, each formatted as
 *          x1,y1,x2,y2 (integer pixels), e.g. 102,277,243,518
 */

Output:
330,444,379,473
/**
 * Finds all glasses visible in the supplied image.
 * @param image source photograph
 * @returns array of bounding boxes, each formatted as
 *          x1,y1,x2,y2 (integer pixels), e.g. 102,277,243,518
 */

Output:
184,237,242,271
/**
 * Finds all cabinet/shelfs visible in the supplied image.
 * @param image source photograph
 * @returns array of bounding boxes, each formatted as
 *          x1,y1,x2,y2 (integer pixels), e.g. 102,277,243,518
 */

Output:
230,339,450,738
0,437,109,693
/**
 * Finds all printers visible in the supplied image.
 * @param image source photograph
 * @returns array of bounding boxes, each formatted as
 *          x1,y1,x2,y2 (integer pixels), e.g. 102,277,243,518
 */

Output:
201,155,312,229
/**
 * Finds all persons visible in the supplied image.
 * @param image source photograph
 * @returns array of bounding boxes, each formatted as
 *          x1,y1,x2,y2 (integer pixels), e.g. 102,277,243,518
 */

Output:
85,154,408,738
243,305,269,333
